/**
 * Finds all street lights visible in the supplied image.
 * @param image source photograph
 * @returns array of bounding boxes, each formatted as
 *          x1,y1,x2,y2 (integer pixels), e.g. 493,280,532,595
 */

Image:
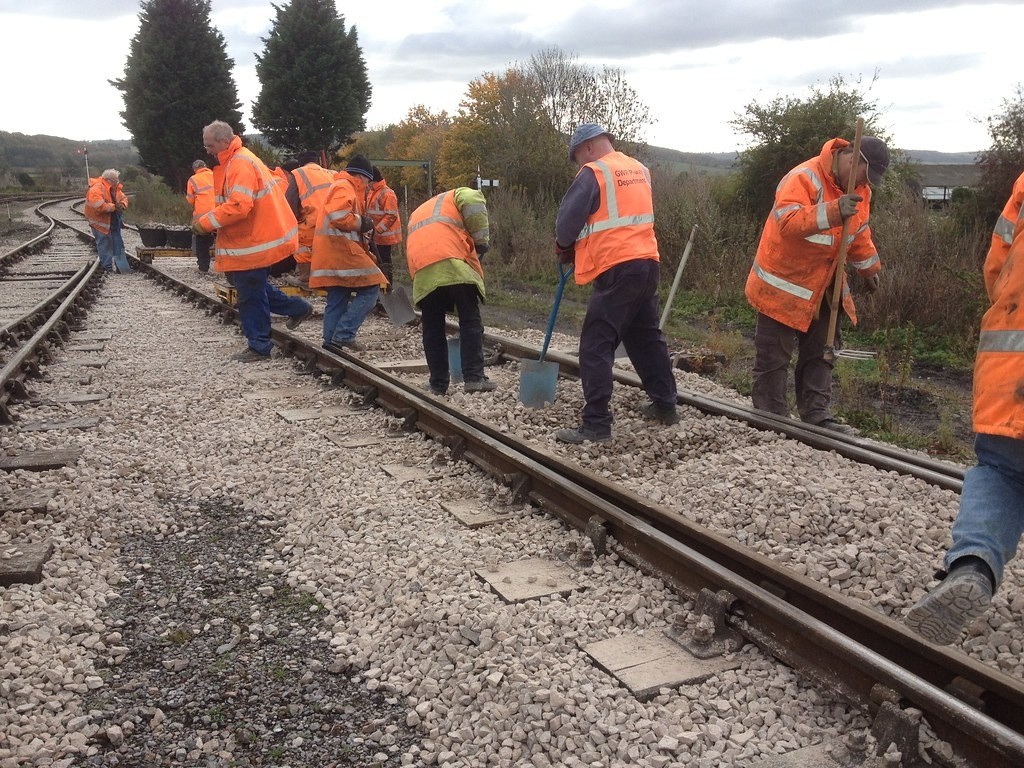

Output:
83,145,90,179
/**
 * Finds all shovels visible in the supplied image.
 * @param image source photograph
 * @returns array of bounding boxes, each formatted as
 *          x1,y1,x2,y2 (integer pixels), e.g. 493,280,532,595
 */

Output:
446,336,464,384
361,226,417,325
522,255,577,408
114,210,132,274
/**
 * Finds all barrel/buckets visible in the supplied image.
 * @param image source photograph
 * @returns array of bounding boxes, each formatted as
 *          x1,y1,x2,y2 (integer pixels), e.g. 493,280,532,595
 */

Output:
137,223,215,249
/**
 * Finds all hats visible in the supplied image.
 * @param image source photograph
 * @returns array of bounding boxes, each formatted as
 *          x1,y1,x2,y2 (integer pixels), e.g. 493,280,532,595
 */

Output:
280,160,299,174
298,151,317,167
192,160,206,168
568,124,615,161
851,135,891,187
370,165,383,182
345,154,373,180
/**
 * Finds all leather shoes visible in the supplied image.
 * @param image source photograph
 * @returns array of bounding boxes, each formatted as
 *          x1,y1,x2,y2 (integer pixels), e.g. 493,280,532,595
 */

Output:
906,560,994,645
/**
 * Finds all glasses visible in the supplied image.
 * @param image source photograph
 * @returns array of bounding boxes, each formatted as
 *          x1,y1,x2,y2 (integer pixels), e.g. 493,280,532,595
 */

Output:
109,178,119,183
204,145,213,150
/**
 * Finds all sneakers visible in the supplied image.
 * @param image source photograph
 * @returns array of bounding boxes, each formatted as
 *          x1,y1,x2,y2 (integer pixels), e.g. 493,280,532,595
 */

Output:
231,348,270,361
332,337,366,351
464,378,498,391
557,426,612,443
641,400,680,425
819,419,854,438
424,380,445,396
286,301,314,330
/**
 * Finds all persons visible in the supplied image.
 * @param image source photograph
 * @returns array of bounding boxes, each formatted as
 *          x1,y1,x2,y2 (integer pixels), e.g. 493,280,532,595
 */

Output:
185,160,215,273
745,136,891,435
84,168,131,274
903,168,1024,647
406,187,498,396
269,151,338,279
366,166,403,295
554,123,681,443
191,119,314,363
308,155,391,352
